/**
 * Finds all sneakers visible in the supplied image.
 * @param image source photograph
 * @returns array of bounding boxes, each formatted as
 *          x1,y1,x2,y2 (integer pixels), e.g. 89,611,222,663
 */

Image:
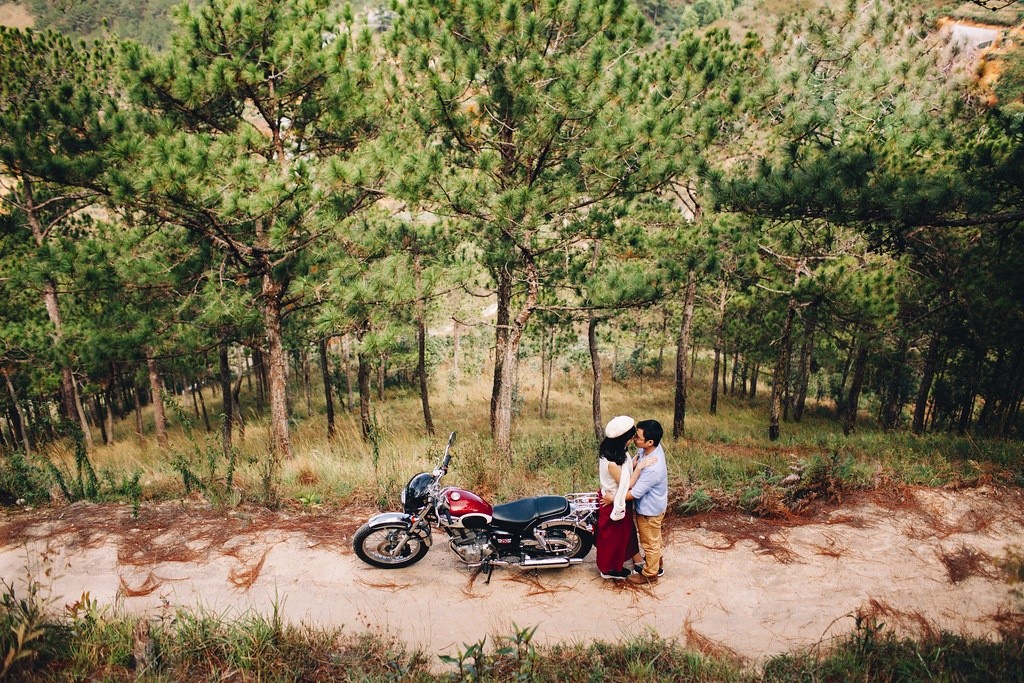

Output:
633,564,664,577
600,567,632,578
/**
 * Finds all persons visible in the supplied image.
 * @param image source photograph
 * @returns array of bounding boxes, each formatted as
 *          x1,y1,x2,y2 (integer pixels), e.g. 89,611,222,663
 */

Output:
594,416,665,580
598,419,667,584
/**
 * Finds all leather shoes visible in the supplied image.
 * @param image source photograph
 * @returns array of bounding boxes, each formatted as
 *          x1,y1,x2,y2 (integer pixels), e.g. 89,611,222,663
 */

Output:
660,557,663,570
627,573,658,584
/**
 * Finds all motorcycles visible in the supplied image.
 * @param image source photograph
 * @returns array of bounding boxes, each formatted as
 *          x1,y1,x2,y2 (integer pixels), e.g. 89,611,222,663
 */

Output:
352,429,602,595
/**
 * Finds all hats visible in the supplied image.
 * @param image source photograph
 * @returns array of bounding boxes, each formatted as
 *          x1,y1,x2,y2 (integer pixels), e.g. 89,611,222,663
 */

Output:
605,416,635,438
